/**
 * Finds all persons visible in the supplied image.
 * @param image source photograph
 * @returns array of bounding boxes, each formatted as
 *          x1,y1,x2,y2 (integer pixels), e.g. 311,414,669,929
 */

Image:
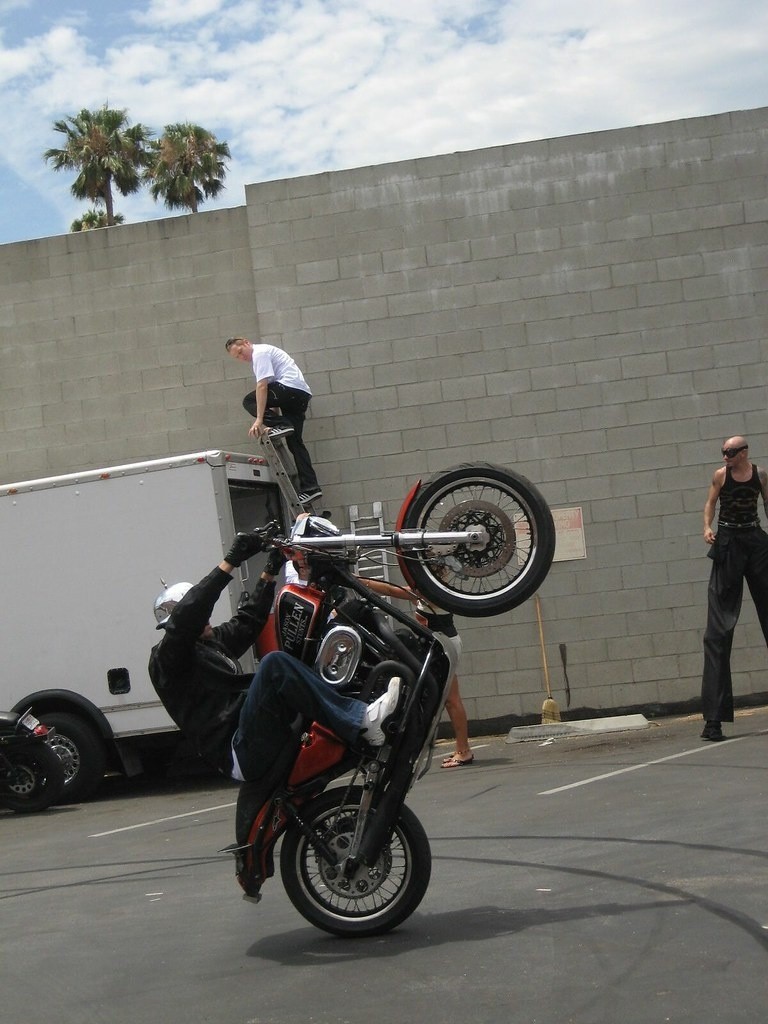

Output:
353,543,475,769
223,335,325,506
701,434,768,738
148,529,403,784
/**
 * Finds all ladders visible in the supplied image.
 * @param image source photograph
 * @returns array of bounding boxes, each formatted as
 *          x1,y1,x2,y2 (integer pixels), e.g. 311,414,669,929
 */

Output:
259,427,317,520
349,501,394,630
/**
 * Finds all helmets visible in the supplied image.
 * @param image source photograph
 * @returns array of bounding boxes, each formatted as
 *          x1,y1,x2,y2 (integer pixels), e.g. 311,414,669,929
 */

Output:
153,577,194,629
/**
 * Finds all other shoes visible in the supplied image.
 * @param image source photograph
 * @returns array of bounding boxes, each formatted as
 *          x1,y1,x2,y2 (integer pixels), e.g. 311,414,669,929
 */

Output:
702,723,722,740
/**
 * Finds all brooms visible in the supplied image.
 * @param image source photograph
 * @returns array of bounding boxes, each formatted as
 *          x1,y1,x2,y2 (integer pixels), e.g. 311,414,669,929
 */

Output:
534,594,560,725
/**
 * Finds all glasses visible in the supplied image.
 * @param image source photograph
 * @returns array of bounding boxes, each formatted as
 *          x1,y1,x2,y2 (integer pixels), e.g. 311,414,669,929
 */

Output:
721,445,749,457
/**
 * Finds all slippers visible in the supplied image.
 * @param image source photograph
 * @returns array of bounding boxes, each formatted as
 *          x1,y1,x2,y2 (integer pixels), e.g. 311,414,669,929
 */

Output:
441,753,474,768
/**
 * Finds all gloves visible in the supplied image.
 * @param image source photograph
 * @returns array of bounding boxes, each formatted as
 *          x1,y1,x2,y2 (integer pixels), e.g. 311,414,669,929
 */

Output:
224,533,263,568
263,537,289,575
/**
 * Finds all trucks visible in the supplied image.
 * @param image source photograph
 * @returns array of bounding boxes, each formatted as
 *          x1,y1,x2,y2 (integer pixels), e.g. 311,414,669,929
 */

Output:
0,449,307,807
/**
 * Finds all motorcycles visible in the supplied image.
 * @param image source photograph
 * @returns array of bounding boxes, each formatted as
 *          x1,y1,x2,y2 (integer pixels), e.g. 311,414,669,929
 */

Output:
222,460,558,938
0,706,65,814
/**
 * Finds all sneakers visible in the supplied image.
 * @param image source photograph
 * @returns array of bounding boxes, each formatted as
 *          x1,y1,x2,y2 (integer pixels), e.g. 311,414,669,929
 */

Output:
298,488,323,504
361,676,404,746
268,427,295,441
